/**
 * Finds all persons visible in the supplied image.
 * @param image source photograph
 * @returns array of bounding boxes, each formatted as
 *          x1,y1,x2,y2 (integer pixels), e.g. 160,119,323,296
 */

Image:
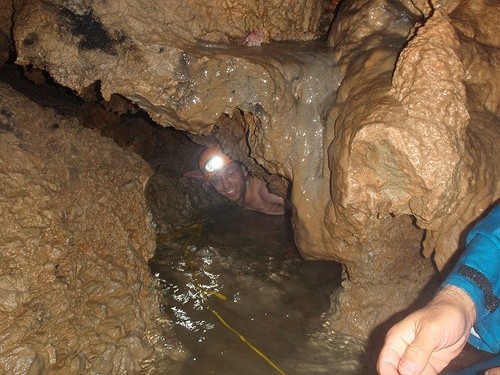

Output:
182,147,292,216
375,203,499,375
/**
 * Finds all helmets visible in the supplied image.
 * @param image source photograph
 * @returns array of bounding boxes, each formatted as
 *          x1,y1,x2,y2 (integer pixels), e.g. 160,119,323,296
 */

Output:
198,148,234,185
